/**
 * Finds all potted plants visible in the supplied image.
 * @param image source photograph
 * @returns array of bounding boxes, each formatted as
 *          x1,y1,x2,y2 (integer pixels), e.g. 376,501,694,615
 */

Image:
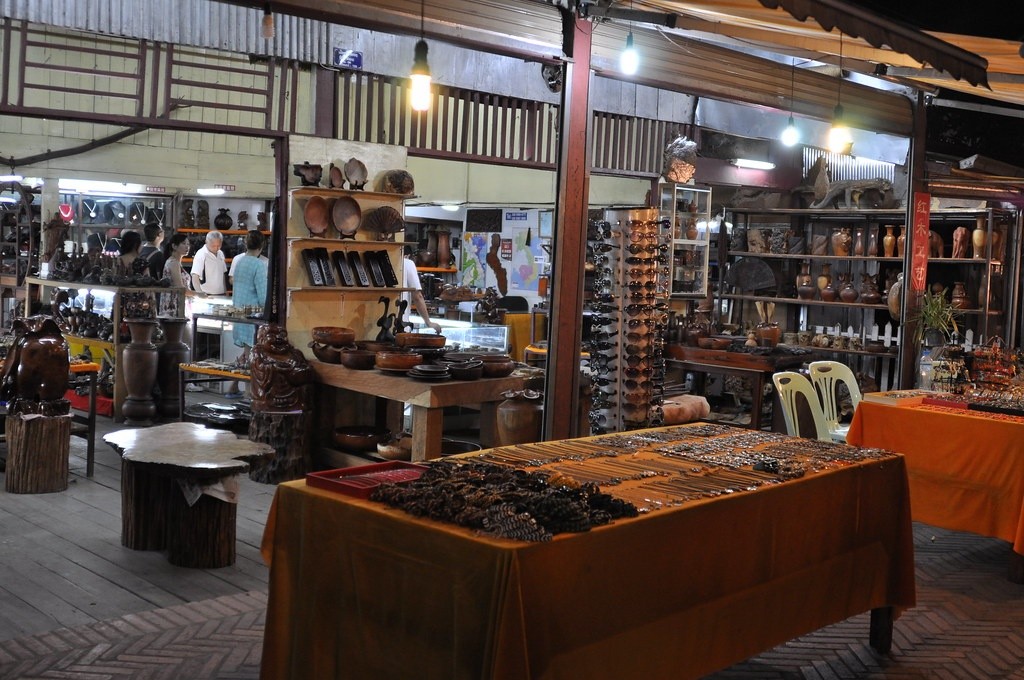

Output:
908,287,958,346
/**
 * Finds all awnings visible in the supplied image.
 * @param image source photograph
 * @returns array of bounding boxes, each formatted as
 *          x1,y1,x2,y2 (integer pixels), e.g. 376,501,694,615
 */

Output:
756,0,994,91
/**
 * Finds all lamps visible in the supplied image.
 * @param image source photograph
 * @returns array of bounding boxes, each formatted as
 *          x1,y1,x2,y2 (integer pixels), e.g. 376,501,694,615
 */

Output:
729,156,775,172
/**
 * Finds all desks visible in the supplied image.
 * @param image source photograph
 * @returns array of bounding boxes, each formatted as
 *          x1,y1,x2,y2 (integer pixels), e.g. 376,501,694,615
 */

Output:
257,422,919,680
0,356,101,478
661,345,826,430
312,360,530,461
845,386,1024,585
104,420,269,566
177,359,253,434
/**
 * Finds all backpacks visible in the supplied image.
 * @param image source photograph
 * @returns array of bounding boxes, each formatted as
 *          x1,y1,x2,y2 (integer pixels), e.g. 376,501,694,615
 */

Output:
130,249,160,277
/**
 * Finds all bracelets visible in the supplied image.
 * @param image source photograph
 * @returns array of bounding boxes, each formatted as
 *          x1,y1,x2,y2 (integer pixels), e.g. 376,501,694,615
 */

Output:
780,466,804,479
371,462,638,543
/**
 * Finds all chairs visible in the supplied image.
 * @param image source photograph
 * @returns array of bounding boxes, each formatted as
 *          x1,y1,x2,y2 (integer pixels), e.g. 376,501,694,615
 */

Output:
772,371,834,442
808,360,864,443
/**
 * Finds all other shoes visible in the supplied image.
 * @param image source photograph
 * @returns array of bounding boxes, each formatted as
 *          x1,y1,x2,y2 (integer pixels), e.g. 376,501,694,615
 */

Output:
183,383,204,392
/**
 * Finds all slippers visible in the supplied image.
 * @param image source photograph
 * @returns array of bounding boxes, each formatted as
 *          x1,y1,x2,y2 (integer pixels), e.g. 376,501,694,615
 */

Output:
225,391,245,399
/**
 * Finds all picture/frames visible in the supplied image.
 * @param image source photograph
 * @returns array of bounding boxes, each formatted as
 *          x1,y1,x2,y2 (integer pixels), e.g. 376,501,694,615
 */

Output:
537,211,554,239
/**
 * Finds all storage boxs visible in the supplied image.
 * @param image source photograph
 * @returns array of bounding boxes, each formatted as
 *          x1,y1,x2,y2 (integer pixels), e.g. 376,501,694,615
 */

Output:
304,460,429,497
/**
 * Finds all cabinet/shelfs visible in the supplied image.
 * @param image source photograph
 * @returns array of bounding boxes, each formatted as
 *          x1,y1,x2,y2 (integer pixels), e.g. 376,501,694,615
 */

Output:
287,186,419,297
654,181,712,296
711,204,1019,360
33,190,174,255
26,275,186,416
176,226,271,265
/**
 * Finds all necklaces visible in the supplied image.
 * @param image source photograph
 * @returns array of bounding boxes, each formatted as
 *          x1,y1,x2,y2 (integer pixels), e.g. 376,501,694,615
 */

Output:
465,439,780,515
136,204,146,224
762,439,902,473
111,203,125,218
334,468,421,488
153,210,165,226
97,233,108,253
58,205,72,218
84,202,96,218
115,239,122,248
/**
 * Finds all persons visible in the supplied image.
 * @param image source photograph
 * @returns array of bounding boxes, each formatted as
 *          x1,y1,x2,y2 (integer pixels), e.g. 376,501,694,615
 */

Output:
163,233,204,392
403,259,442,335
225,230,268,402
191,231,227,386
86,224,164,302
